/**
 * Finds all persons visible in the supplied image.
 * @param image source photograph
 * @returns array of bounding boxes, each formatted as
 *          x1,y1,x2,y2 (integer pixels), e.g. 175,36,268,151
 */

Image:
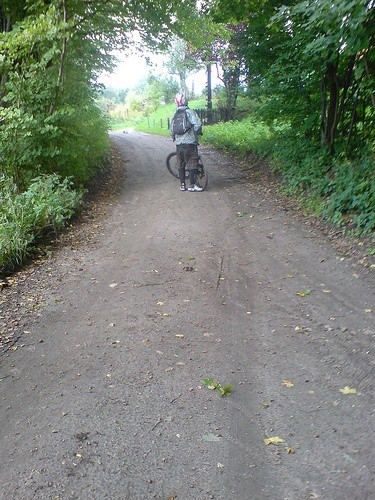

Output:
170,93,204,192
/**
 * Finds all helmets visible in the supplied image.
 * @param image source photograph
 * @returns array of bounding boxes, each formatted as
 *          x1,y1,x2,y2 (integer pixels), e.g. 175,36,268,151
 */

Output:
175,94,188,107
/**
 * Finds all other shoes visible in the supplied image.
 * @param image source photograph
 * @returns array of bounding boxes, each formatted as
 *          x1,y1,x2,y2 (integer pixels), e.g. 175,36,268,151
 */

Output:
180,185,185,190
187,183,203,191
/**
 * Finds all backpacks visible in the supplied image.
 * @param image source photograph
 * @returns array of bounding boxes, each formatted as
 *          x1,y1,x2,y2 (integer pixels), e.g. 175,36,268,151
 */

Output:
171,107,194,136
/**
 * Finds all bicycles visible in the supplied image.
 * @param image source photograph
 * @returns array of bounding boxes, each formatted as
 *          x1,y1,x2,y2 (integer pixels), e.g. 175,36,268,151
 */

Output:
165,140,210,190
